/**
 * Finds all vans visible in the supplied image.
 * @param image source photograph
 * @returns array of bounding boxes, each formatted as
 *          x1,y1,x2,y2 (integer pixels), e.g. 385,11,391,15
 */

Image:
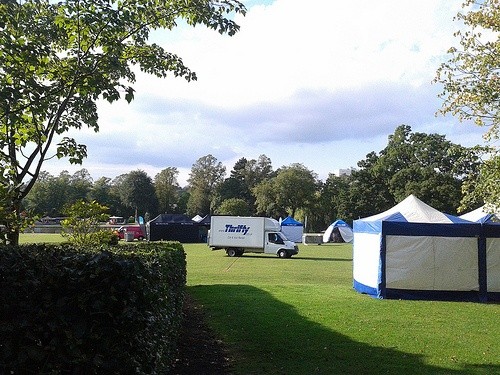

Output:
116,225,146,241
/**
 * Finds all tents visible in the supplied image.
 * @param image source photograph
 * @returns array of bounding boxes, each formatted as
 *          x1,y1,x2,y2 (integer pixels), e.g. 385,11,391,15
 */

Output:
146,214,210,243
352,194,500,304
323,219,353,243
281,216,303,242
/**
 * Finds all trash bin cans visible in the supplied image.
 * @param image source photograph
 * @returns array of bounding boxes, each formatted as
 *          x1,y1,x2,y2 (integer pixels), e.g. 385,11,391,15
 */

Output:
124,231,134,242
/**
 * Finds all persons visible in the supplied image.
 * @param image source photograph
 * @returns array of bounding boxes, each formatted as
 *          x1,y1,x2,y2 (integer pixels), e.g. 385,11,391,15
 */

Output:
120,224,125,232
91,220,97,225
110,219,118,232
333,231,339,242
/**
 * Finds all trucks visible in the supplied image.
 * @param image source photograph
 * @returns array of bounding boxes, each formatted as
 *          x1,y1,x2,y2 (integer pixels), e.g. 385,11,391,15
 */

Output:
207,216,299,259
